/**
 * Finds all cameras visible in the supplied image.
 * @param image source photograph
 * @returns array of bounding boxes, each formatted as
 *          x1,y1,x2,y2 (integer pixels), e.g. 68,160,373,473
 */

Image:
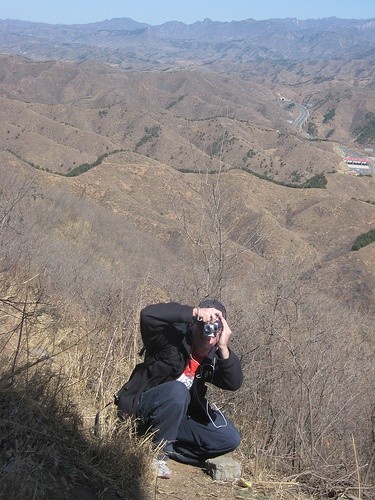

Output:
202,320,222,338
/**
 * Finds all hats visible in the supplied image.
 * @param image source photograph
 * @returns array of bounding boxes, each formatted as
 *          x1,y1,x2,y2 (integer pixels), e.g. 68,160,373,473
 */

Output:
198,300,226,322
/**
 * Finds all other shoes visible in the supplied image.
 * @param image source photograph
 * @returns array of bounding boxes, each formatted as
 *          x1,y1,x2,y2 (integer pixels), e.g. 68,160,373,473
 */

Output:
152,459,172,478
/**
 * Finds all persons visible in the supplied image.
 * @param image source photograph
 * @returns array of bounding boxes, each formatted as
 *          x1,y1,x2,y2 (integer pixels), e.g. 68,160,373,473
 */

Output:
114,299,244,479
